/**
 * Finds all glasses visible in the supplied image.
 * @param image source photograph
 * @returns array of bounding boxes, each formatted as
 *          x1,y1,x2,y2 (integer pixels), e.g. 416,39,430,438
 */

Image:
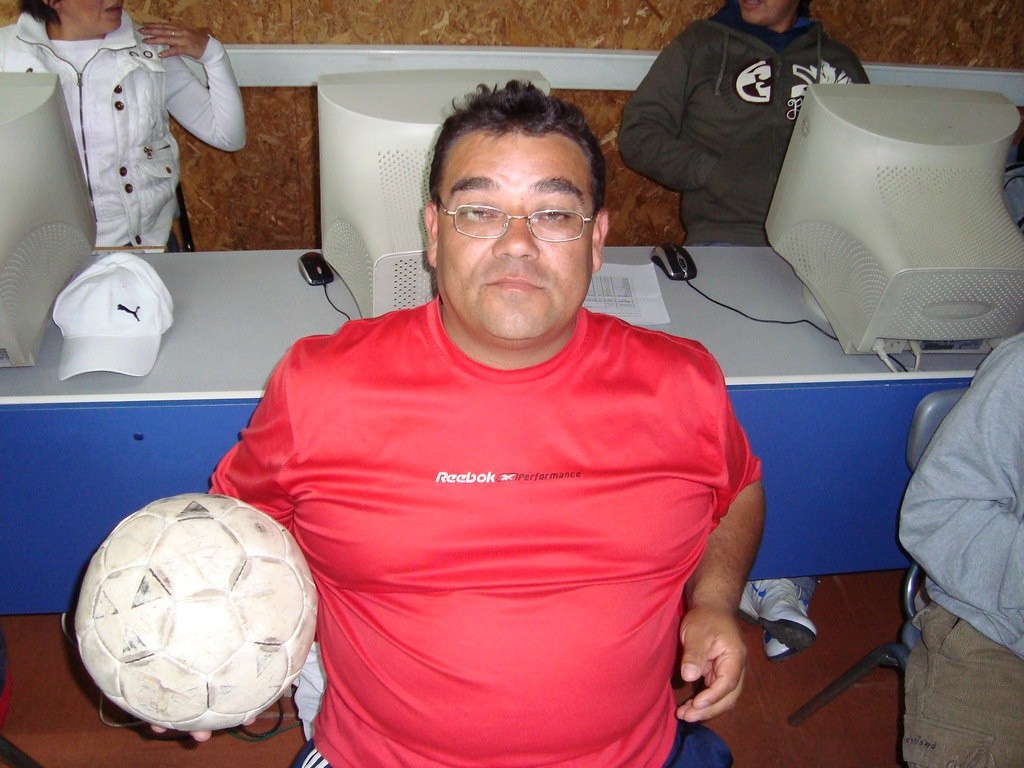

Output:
437,201,596,243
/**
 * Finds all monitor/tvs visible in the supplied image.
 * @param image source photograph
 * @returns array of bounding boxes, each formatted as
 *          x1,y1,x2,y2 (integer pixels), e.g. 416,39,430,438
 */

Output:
0,72,97,368
765,84,1024,355
317,71,550,318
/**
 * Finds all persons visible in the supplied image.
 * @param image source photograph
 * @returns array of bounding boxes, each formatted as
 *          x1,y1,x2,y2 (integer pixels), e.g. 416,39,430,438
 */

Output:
150,81,766,768
616,0,871,660
1,0,246,255
898,332,1024,768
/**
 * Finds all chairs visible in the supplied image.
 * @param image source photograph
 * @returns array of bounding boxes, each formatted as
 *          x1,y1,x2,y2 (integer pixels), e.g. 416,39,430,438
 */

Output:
786,387,970,727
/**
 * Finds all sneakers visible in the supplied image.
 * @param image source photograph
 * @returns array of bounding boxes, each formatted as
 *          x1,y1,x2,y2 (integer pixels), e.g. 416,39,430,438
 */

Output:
738,577,816,650
762,598,810,662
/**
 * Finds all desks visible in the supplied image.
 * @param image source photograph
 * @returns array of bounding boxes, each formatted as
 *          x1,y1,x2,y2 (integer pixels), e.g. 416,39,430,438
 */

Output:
0,248,997,616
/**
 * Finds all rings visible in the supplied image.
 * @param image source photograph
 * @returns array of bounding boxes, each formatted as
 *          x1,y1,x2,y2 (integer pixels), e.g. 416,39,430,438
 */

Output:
173,30,174,37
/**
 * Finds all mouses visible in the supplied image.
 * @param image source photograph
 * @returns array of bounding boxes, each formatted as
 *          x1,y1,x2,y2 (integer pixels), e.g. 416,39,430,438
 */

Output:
299,252,334,286
650,241,697,281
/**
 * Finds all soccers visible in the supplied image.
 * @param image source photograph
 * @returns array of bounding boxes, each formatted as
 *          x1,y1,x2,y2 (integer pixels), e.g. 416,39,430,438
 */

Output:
73,493,318,732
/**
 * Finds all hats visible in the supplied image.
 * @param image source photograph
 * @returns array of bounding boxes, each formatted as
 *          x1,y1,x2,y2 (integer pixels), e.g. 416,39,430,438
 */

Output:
52,252,175,381
